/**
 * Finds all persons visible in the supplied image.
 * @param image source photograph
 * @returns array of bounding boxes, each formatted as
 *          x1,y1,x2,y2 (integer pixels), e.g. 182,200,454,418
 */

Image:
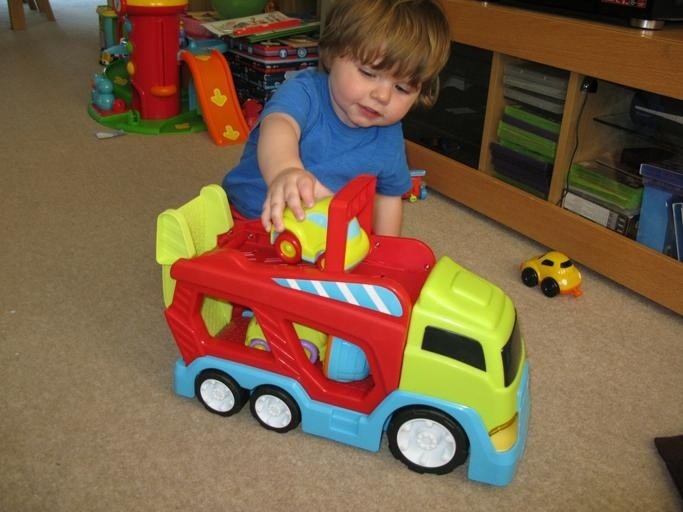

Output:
222,1,452,238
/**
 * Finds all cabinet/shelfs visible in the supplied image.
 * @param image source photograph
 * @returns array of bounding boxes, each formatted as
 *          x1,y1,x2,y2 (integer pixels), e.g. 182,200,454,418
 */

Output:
405,0,683,318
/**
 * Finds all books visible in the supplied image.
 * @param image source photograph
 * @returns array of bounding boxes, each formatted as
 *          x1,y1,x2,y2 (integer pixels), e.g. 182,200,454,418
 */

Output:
201,8,320,44
